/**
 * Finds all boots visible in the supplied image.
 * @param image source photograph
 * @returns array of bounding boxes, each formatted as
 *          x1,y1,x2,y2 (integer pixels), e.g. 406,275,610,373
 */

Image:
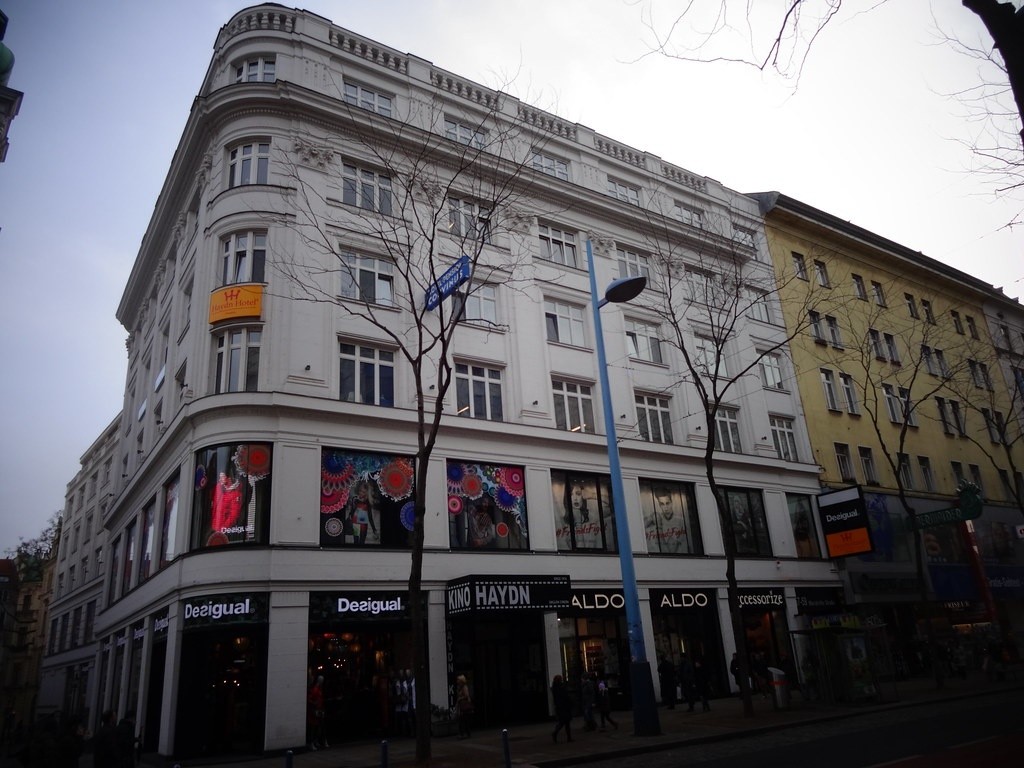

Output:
706,704,711,711
703,706,706,712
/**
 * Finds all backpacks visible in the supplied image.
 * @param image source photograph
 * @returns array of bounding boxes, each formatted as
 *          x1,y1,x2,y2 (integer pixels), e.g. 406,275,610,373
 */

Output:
730,659,738,674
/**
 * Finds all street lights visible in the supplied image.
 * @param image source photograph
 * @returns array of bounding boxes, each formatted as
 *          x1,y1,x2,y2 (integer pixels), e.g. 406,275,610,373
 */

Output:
584,236,665,737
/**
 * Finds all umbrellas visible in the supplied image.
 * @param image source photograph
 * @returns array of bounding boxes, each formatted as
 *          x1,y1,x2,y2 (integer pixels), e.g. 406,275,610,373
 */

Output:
137,727,142,765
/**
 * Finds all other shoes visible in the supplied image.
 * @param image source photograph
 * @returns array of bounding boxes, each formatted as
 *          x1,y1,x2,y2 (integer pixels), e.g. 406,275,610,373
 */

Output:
686,706,694,713
744,707,754,714
551,731,558,744
459,737,464,740
599,725,606,728
465,736,471,739
567,738,576,743
614,720,619,730
583,725,595,732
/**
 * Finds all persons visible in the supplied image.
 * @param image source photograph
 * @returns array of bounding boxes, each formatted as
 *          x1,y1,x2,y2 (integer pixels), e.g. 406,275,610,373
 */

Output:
395,668,416,738
730,647,753,705
551,674,576,743
929,637,1019,694
307,674,331,751
679,652,714,712
455,674,472,740
2,710,142,768
657,655,678,709
582,677,619,732
801,647,822,704
769,650,794,712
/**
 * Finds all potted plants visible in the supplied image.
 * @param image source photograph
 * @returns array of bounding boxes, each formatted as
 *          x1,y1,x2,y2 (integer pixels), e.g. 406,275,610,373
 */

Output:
429,701,459,737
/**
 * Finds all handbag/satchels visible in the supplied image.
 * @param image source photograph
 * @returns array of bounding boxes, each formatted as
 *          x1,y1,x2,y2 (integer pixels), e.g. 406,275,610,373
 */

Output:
314,710,325,721
461,699,483,717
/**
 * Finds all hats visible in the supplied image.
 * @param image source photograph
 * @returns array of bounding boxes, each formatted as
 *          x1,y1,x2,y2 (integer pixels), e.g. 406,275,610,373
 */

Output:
458,674,466,683
599,680,605,696
